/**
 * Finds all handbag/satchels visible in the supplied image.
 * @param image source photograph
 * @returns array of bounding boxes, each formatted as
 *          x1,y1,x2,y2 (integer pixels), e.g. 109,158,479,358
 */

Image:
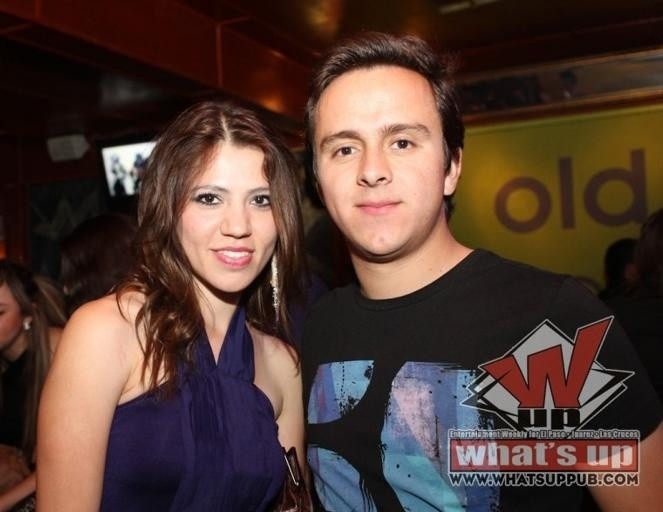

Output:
274,446,315,512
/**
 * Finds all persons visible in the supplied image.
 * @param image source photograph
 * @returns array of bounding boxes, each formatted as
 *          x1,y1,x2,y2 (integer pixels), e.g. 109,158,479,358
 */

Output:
32,102,312,511
111,157,128,198
0,211,330,511
610,209,662,405
287,28,662,511
593,237,643,301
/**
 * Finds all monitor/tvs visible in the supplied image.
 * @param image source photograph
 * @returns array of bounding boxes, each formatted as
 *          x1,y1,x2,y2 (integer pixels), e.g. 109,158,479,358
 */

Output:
97,135,156,205
